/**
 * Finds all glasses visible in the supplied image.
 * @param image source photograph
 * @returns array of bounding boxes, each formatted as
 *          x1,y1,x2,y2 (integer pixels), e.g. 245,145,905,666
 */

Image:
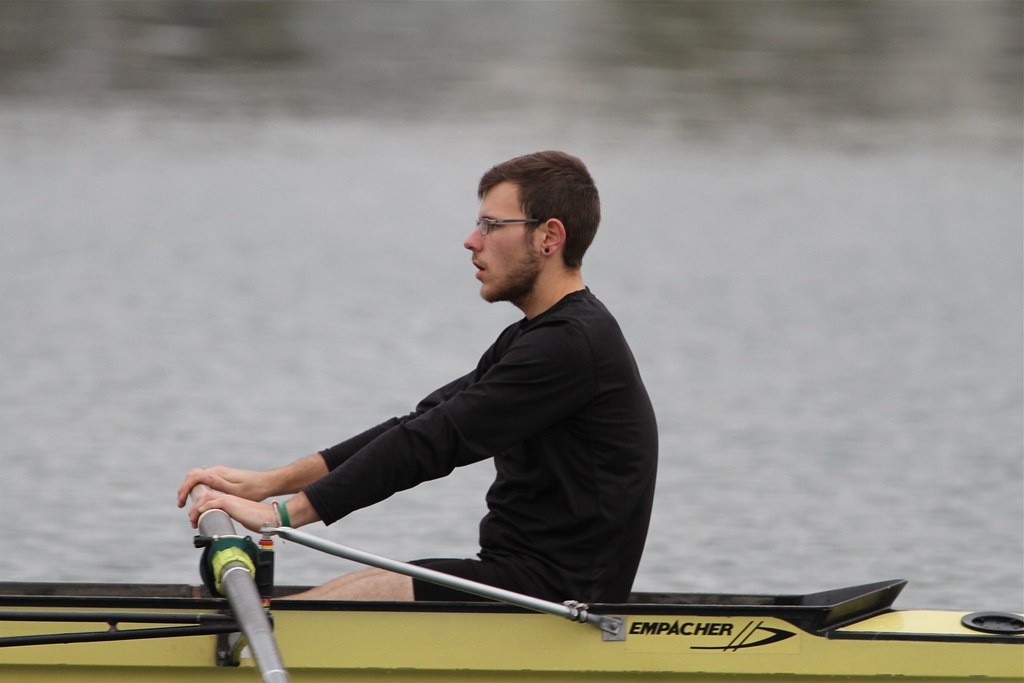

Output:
476,217,539,236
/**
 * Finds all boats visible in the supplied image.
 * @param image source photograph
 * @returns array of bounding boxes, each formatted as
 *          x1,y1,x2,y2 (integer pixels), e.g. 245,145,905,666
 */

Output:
0,577,1024,683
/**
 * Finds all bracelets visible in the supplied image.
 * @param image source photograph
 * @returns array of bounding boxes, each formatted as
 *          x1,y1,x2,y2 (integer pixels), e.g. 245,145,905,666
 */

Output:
270,500,291,528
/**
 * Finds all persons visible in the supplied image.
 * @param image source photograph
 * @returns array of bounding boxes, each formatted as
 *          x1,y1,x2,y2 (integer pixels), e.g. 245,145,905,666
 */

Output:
175,150,659,602
